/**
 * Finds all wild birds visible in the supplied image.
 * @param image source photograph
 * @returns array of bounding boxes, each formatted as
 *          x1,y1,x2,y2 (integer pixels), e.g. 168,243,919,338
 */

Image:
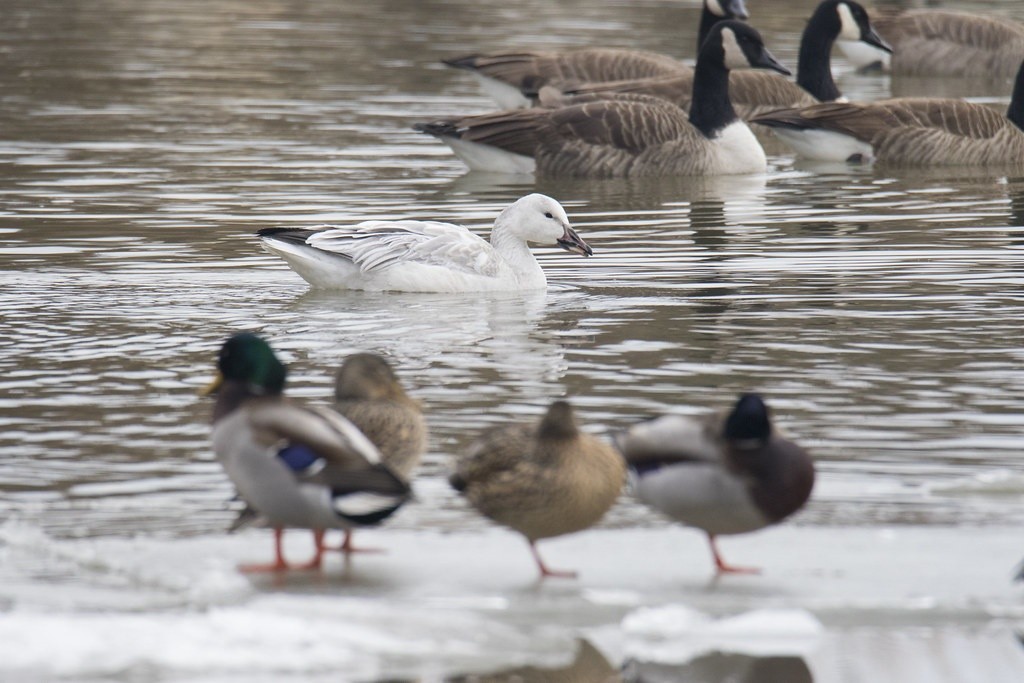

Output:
224,354,428,554
445,401,625,573
255,193,592,291
412,0,1024,174
618,393,816,577
197,333,421,572
